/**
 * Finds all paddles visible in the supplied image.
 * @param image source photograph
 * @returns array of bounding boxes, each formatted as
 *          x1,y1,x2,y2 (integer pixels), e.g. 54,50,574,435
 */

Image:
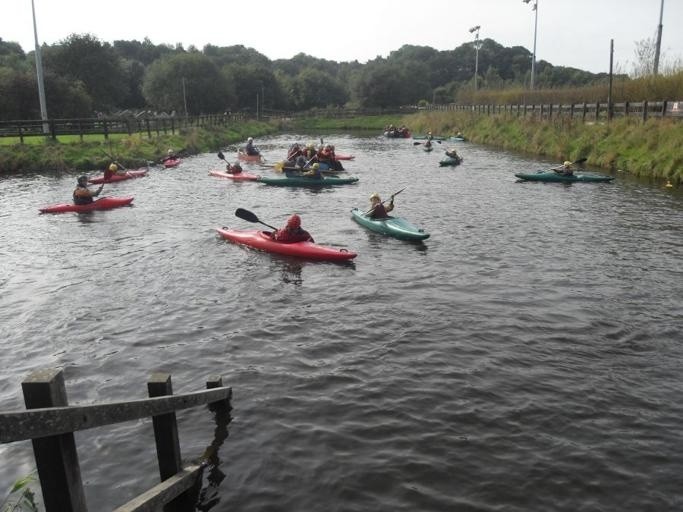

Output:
235,208,278,230
414,141,425,145
149,149,186,166
99,169,114,186
217,152,231,169
360,187,407,216
540,157,587,174
273,148,300,172
437,141,447,150
100,149,137,179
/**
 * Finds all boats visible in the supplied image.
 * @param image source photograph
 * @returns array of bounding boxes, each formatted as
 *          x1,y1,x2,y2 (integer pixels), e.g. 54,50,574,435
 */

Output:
163,156,181,168
514,175,614,182
413,135,465,153
210,169,260,180
39,195,134,214
352,208,429,242
260,174,358,187
439,153,461,166
277,155,357,174
384,130,410,138
237,149,266,162
218,226,357,261
88,170,146,185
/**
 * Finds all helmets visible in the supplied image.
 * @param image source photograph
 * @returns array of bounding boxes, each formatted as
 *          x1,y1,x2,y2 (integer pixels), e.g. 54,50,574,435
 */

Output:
165,136,335,170
76,174,90,188
563,161,571,166
368,192,381,204
286,213,301,227
108,163,118,172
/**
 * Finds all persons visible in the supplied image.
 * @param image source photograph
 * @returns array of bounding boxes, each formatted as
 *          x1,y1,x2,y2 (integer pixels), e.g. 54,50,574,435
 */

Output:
455,132,463,138
270,213,314,244
247,136,260,158
422,132,434,147
384,124,410,138
281,141,339,179
225,163,242,175
445,148,463,161
364,192,395,218
161,149,178,164
550,161,574,176
105,162,125,179
72,175,102,203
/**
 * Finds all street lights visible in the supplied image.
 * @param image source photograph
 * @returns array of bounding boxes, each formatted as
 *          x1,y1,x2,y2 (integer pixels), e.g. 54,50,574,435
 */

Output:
523,0,538,84
469,25,480,89
